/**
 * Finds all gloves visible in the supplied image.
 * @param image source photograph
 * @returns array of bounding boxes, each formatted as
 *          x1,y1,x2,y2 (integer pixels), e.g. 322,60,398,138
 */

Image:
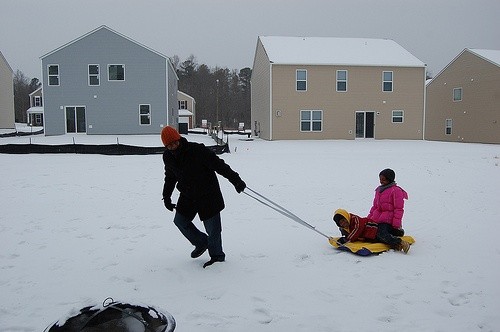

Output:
336,237,348,245
234,178,246,193
390,228,399,236
163,196,176,211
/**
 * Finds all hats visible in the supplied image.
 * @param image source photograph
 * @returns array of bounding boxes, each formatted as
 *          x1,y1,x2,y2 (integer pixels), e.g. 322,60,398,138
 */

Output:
162,126,180,146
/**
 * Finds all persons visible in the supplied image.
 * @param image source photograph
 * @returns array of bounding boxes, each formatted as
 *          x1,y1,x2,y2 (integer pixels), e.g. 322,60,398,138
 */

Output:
333,209,410,254
161,126,246,268
367,168,408,236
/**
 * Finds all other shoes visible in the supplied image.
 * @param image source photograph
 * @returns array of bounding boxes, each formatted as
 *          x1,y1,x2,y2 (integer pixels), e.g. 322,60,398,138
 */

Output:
394,244,402,251
203,260,214,269
191,236,208,258
401,240,409,253
